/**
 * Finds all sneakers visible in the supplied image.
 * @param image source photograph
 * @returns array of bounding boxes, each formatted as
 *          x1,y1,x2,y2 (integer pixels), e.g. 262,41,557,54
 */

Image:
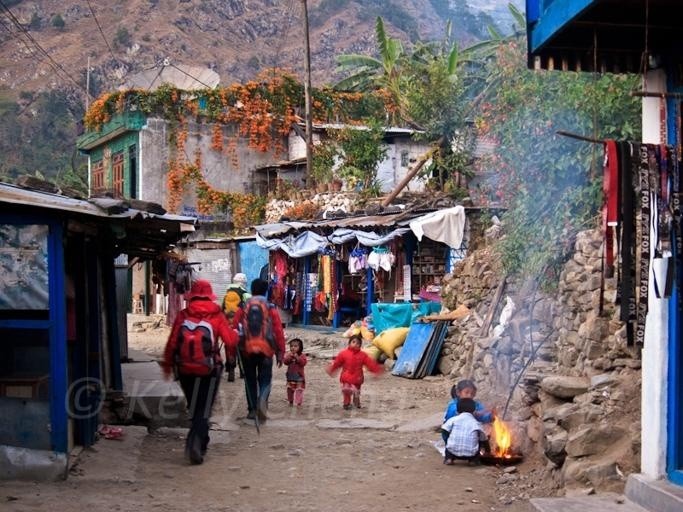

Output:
186,426,211,465
247,399,271,421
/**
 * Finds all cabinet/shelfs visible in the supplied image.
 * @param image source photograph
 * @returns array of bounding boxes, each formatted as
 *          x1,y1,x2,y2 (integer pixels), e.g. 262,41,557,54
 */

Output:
412,254,447,294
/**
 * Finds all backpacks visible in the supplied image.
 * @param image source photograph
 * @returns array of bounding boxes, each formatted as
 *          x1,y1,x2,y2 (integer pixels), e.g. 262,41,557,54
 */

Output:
172,308,220,378
222,285,245,324
241,299,275,359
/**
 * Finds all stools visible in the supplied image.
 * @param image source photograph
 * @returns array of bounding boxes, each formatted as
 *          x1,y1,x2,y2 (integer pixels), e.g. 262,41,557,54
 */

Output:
133,299,144,314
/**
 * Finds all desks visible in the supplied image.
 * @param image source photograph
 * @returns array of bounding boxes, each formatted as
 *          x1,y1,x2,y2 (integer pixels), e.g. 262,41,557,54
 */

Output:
0,373,49,402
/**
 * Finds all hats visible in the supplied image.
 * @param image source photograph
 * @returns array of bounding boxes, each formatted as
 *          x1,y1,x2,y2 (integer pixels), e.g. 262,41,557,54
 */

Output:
232,273,247,286
183,278,218,301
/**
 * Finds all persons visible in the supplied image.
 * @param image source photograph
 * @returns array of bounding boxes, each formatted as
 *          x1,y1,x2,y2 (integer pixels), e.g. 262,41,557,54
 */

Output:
327,334,382,411
162,273,307,462
432,381,496,465
